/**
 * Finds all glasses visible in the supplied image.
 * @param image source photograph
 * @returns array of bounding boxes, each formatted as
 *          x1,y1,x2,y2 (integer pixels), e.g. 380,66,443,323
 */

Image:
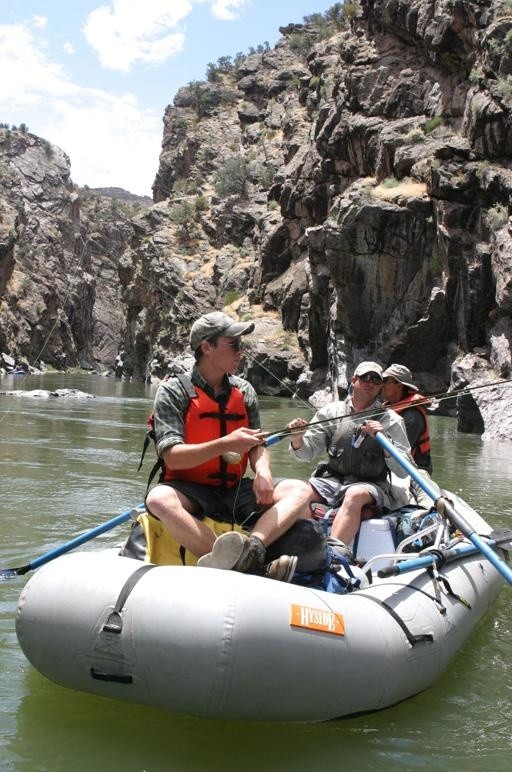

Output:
210,340,244,350
357,374,381,384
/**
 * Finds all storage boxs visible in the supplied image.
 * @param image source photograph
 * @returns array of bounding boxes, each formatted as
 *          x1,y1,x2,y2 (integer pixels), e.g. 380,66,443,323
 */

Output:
350,517,397,573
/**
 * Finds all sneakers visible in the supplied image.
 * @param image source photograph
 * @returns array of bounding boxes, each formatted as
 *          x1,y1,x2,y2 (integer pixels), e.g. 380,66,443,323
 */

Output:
263,554,298,582
196,531,264,570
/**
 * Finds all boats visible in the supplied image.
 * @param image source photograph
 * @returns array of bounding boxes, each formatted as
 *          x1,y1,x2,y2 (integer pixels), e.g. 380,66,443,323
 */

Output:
14,490,506,722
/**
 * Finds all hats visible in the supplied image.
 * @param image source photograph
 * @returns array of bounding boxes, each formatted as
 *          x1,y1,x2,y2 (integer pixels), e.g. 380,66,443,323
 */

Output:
382,363,419,393
189,311,255,350
354,361,383,381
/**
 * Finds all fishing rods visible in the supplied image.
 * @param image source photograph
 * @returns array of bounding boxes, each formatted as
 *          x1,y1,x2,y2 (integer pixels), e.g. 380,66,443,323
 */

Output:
89,237,320,414
222,379,512,441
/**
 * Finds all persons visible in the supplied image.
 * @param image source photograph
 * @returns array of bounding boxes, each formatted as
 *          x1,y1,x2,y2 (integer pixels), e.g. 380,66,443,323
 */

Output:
368,364,433,485
282,360,417,557
143,311,316,588
